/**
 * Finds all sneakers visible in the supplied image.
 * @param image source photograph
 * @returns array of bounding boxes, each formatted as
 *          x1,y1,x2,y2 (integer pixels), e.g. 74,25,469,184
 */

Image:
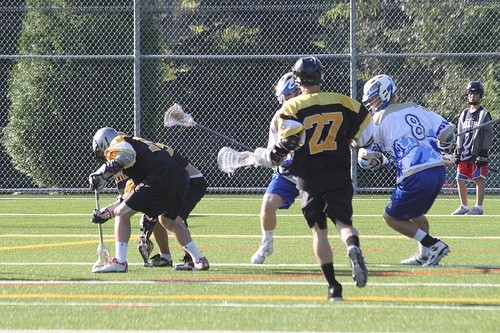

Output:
347,244,369,288
400,251,429,266
193,256,210,271
451,205,470,215
143,253,174,268
328,283,343,301
465,206,483,215
95,256,128,273
421,239,451,267
251,241,274,264
174,252,195,272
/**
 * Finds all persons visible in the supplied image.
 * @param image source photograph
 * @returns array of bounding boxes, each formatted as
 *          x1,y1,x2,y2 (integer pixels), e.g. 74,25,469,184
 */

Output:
252,72,303,264
451,81,494,215
250,58,377,302
89,128,210,274
358,75,455,267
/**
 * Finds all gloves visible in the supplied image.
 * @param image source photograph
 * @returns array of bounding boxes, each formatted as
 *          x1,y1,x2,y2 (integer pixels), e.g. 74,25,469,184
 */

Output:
91,197,124,224
475,151,490,167
253,146,285,169
357,147,390,171
452,153,461,166
88,163,114,192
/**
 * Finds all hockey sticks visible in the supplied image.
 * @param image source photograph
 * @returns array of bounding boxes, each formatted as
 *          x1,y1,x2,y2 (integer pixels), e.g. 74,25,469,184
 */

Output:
92,177,112,272
456,117,500,136
216,146,258,173
163,102,255,151
442,153,499,173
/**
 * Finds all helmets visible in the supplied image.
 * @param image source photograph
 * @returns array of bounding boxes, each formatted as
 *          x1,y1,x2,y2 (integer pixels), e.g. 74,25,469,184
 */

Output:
291,57,326,89
91,127,119,161
465,80,484,99
275,71,301,106
361,74,400,116
